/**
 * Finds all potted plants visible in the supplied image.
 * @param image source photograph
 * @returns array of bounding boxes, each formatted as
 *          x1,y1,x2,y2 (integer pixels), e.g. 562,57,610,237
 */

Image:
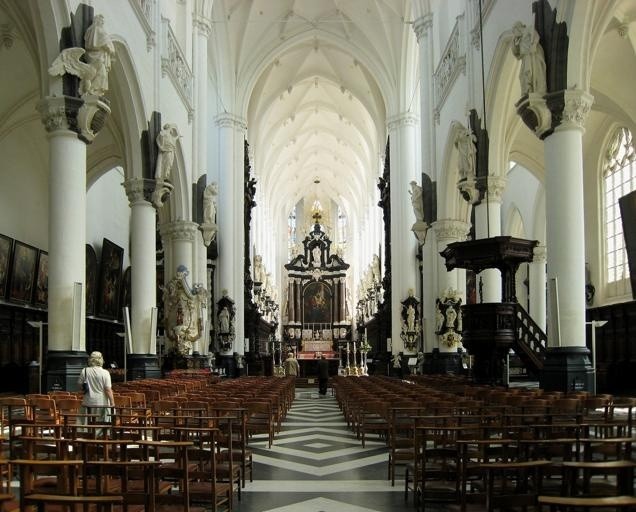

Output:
0,233,15,299
86,242,98,315
7,238,41,305
123,261,133,311
33,250,64,308
99,236,125,321
301,282,334,326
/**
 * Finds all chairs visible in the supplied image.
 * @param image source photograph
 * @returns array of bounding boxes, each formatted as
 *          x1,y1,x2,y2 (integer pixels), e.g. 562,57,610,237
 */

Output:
2,361,635,512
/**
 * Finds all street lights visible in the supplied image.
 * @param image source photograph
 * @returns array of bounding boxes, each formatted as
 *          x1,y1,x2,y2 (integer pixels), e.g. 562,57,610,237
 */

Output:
81,381,89,396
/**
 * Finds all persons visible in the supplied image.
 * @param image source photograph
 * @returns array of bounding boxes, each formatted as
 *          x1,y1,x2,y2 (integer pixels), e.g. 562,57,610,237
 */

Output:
75,351,116,436
316,356,328,395
285,352,301,377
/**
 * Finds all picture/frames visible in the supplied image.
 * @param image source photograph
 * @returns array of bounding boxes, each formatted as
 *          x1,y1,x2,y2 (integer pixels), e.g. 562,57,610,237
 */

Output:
0,233,15,299
7,238,41,305
33,250,64,308
99,236,125,321
123,261,133,311
86,242,98,315
301,282,334,326
